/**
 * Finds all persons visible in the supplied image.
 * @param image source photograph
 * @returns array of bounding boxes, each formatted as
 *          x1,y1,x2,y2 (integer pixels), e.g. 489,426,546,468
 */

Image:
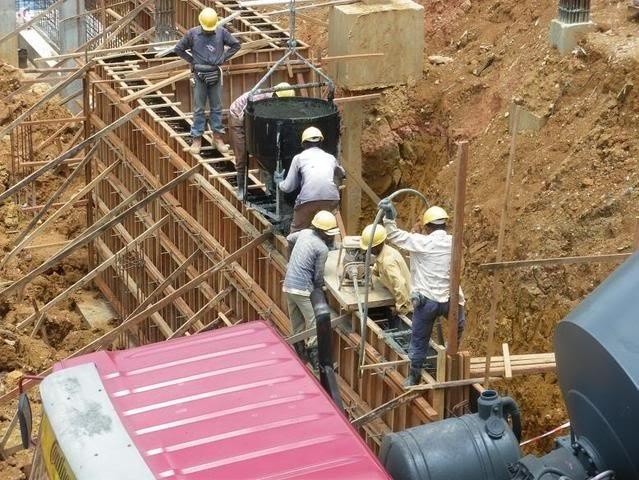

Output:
282,210,340,369
378,199,466,390
360,223,414,331
273,125,346,242
174,7,241,154
228,82,296,201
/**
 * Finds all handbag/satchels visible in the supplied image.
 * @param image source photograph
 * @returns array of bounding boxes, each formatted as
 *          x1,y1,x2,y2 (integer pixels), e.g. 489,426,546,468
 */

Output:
197,70,219,88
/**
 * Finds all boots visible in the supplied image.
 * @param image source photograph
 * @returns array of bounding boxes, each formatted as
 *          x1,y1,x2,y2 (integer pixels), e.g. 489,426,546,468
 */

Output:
190,137,201,153
212,133,228,153
402,367,421,386
294,339,338,372
236,160,248,201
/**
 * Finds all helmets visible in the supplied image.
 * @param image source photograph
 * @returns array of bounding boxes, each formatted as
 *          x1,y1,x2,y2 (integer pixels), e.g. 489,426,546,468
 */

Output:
423,206,449,226
311,210,340,236
275,82,295,98
198,6,219,31
302,126,324,143
359,224,387,250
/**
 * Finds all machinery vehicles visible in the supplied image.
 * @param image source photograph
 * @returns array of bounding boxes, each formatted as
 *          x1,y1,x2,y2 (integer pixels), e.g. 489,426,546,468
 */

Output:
16,238,639,480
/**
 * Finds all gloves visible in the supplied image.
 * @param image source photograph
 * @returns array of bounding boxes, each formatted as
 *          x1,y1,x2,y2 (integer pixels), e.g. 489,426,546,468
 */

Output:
379,197,397,219
274,168,286,184
